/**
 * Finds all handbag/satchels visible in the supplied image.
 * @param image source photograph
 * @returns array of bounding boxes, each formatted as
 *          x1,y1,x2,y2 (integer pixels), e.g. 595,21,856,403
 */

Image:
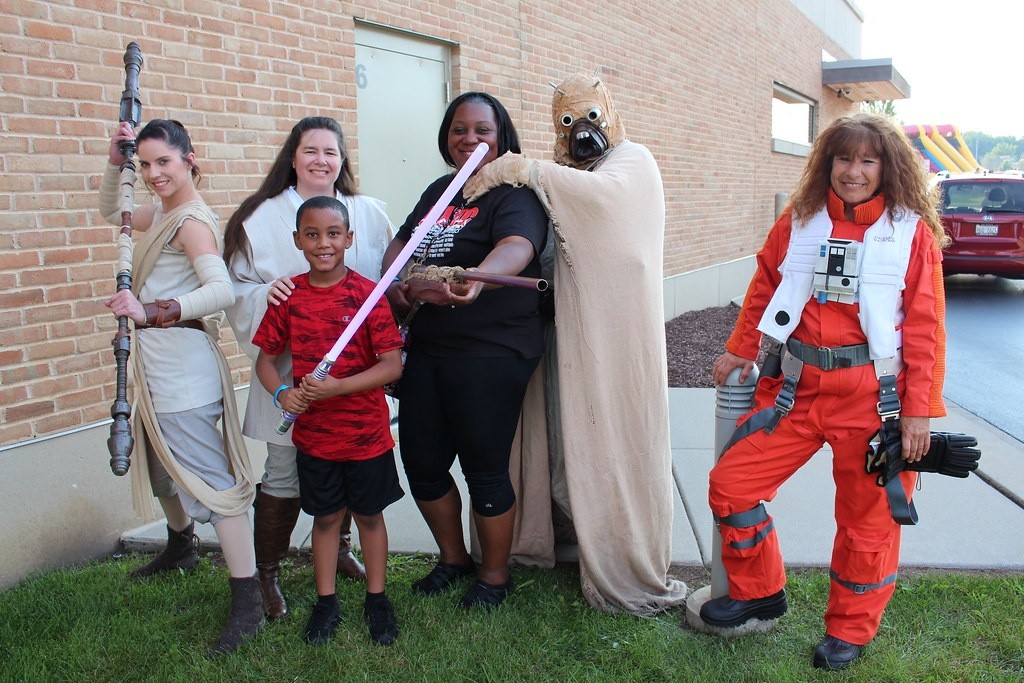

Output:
379,325,409,396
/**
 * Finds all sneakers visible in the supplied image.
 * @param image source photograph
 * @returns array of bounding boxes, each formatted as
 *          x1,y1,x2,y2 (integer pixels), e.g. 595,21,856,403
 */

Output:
458,574,514,612
366,600,397,645
412,554,475,595
699,588,788,628
813,635,860,670
304,602,340,646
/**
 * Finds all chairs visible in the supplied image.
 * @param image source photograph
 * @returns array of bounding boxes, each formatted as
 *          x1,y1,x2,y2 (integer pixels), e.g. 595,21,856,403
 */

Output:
980,188,1007,213
940,192,956,214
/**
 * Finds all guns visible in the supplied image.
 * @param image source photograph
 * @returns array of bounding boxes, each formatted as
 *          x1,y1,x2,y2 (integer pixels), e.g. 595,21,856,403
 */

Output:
404,264,549,303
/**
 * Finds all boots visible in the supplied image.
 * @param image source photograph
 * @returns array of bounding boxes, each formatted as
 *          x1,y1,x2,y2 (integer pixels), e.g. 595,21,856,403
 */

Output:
252,483,302,619
216,575,264,658
133,518,201,576
336,508,367,581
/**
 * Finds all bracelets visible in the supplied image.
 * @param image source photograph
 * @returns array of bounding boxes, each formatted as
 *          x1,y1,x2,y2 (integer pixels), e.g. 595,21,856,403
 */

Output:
274,385,291,408
394,277,399,280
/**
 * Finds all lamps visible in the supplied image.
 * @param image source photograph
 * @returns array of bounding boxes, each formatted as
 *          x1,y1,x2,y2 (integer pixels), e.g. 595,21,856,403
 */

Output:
837,88,844,98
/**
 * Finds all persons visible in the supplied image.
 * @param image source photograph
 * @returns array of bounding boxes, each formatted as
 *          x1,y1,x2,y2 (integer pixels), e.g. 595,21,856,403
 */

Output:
700,114,948,671
223,117,394,620
463,67,686,616
252,196,404,645
382,91,556,611
99,119,267,657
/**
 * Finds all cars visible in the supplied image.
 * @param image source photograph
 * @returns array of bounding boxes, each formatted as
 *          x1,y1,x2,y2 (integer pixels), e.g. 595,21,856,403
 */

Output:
932,170,1024,280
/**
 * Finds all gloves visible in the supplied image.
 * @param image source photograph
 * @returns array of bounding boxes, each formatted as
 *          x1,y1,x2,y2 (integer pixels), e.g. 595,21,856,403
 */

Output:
463,149,527,204
864,428,982,486
